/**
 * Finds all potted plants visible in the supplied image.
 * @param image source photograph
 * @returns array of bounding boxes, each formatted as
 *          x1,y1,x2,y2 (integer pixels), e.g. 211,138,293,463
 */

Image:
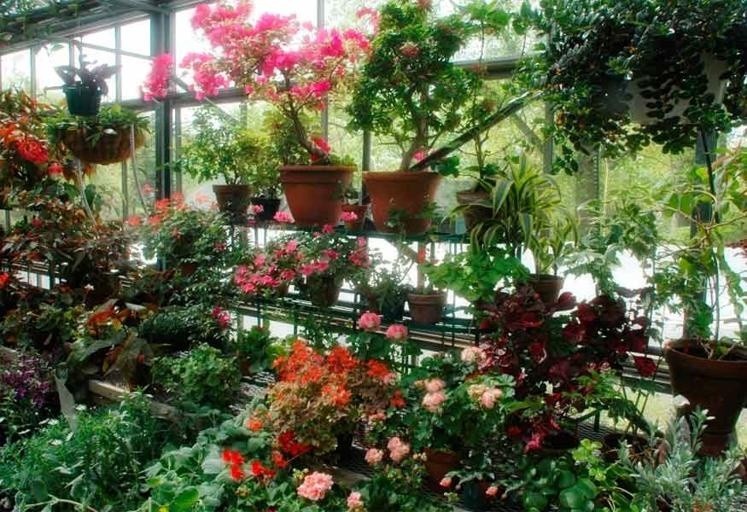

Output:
1,1,747,512
